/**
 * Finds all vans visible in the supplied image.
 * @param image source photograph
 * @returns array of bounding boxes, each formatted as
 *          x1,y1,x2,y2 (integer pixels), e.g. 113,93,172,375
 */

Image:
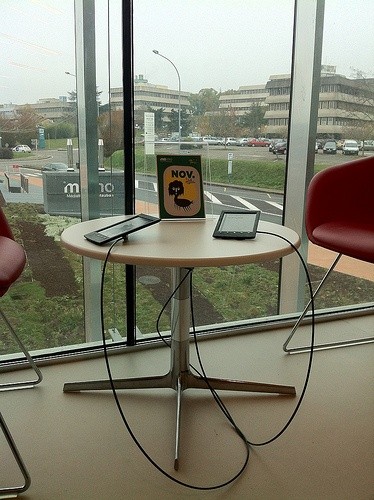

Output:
341,140,361,156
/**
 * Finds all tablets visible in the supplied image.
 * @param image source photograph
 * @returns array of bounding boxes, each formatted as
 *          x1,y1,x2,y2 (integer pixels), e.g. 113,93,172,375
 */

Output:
213,210,260,239
85,213,161,243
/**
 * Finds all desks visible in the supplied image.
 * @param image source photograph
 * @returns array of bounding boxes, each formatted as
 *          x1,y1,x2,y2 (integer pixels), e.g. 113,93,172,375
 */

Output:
61,213,301,472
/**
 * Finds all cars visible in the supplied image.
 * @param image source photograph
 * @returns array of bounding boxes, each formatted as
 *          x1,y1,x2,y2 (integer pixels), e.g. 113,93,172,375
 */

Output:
323,141,338,154
160,133,374,155
11,145,32,154
41,162,68,174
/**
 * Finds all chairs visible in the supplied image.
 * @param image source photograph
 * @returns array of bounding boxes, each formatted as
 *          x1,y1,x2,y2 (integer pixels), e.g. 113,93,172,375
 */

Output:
284,156,374,353
0,206,42,500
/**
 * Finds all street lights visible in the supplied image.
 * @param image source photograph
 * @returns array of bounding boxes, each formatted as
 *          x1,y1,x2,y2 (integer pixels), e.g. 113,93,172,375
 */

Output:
153,49,181,141
